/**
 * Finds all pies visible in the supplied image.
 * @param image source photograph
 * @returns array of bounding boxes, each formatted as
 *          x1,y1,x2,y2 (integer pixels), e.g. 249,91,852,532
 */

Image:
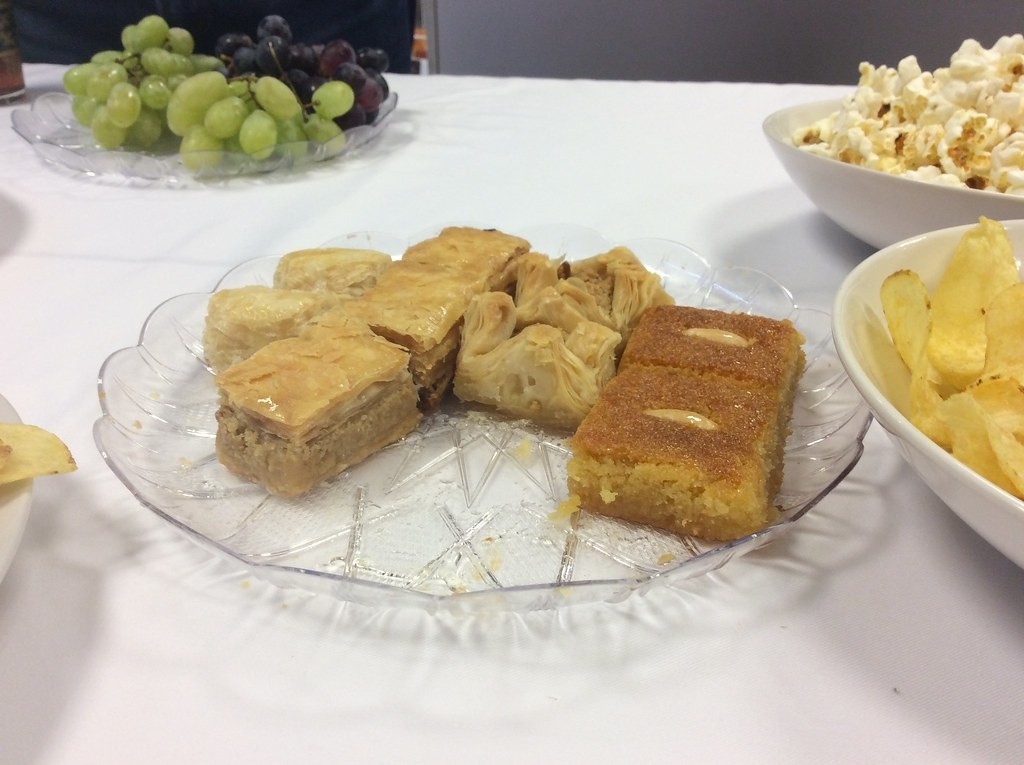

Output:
205,224,803,538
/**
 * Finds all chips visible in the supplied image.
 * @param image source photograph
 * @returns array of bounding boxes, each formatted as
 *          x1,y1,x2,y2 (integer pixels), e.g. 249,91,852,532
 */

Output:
881,218,1024,500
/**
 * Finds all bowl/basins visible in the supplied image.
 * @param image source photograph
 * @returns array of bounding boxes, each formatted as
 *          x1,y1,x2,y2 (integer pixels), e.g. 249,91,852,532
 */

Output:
832,217,1024,572
761,99,1024,251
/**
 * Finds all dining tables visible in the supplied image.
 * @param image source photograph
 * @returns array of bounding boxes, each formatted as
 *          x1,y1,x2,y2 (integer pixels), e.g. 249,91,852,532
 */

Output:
0,64,1024,765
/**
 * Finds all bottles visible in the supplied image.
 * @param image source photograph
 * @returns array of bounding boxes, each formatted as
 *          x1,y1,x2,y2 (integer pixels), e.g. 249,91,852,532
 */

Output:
0,9,25,108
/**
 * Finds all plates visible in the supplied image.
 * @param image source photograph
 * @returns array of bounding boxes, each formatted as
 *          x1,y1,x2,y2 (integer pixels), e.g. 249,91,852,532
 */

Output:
7,86,396,178
0,393,30,585
88,232,878,607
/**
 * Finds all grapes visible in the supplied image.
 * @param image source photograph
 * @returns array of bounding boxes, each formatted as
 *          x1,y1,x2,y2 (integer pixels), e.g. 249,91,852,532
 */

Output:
217,15,389,126
64,16,355,171
